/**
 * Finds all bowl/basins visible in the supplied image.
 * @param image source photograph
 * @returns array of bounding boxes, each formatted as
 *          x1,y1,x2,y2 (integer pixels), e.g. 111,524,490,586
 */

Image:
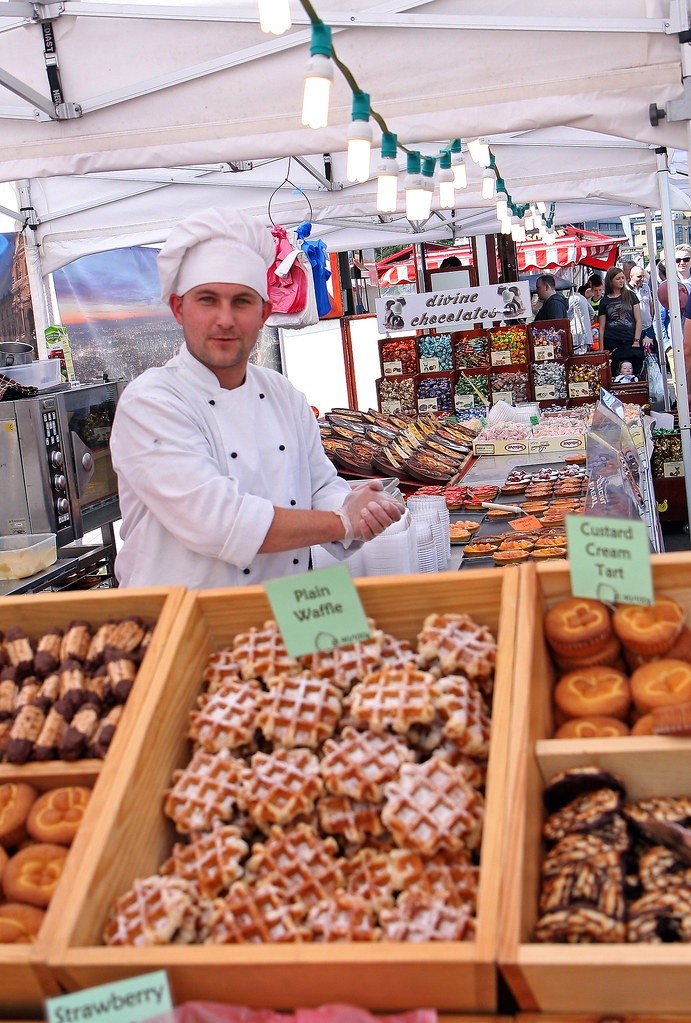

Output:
0,342,34,367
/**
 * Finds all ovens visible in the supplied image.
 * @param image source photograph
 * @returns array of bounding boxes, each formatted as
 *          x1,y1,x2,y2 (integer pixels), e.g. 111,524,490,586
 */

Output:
0,379,131,549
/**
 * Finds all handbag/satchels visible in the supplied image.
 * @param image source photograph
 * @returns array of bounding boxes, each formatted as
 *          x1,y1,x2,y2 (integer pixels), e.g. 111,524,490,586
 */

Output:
639,345,664,412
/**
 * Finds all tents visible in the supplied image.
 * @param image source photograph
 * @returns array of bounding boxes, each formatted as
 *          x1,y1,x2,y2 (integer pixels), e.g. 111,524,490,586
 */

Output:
0,0,691,523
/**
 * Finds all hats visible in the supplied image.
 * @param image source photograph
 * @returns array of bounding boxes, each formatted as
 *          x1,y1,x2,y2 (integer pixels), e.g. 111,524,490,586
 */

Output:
155,204,277,306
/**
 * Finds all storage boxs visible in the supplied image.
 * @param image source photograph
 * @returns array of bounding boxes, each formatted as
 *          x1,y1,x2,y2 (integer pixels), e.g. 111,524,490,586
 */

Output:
0,585,188,1016
45,562,520,1016
0,532,58,581
494,549,691,1013
346,477,407,507
0,358,62,391
374,318,651,456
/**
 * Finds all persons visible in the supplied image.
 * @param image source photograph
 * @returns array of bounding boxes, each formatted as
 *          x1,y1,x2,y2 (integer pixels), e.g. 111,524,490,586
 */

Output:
658,280,691,311
533,274,569,324
597,267,642,353
570,274,604,353
106,205,405,590
653,244,691,357
683,291,691,412
440,256,462,268
623,258,672,375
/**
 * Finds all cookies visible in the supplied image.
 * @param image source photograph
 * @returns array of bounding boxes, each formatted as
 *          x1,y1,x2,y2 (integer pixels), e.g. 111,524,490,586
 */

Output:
530,767,691,945
101,613,495,942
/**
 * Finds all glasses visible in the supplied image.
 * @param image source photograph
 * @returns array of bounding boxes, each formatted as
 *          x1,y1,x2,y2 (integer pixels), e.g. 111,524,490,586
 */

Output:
675,256,691,263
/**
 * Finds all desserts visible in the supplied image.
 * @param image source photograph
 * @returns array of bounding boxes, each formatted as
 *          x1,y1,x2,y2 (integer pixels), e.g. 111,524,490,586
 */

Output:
544,593,691,739
0,783,92,945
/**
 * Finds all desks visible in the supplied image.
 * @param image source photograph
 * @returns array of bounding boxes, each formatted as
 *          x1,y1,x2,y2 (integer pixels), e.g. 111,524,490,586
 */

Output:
438,448,664,572
335,455,479,500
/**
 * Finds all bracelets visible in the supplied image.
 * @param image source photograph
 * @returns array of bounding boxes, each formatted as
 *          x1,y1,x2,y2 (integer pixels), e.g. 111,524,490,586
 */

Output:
634,337,640,342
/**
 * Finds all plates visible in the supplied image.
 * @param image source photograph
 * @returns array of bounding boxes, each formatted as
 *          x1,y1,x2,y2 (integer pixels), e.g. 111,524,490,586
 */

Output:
340,494,452,579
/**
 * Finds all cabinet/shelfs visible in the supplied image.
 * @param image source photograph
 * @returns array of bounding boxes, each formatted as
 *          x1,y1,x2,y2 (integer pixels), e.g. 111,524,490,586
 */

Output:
650,434,689,534
